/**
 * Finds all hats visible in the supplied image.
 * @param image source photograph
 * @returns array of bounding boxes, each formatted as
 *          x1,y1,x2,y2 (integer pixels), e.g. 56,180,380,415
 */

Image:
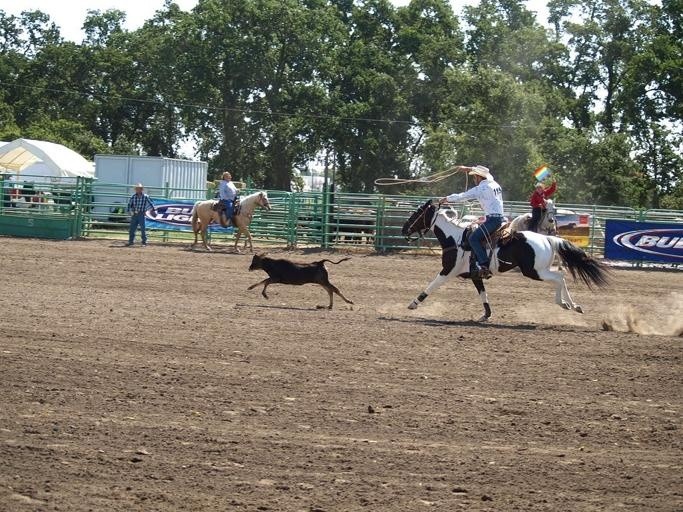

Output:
134,183,145,191
468,165,493,179
534,182,545,189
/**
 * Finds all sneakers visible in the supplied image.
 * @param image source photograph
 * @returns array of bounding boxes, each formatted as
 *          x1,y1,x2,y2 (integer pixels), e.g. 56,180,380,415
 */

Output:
126,241,147,247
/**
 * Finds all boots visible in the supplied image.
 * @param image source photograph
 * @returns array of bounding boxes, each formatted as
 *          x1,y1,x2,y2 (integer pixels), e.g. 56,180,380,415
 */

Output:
480,261,494,278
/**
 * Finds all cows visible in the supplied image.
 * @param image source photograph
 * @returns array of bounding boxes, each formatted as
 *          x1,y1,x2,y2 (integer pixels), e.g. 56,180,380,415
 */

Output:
246,252,354,310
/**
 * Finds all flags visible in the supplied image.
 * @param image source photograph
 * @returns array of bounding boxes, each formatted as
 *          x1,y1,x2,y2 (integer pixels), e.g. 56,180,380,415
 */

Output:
532,164,550,183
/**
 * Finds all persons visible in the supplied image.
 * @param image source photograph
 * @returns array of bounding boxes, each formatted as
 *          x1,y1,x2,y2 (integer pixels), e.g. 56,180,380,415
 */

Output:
526,176,558,232
32,190,45,203
0,171,6,207
124,183,157,248
219,171,239,226
6,174,17,207
437,164,503,280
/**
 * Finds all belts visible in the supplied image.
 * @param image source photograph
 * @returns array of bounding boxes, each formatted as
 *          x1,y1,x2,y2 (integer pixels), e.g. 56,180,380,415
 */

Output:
487,215,504,217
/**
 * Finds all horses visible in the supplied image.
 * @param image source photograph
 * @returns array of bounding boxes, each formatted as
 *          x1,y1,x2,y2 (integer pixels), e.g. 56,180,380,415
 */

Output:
188,188,272,253
508,197,557,236
401,199,612,323
